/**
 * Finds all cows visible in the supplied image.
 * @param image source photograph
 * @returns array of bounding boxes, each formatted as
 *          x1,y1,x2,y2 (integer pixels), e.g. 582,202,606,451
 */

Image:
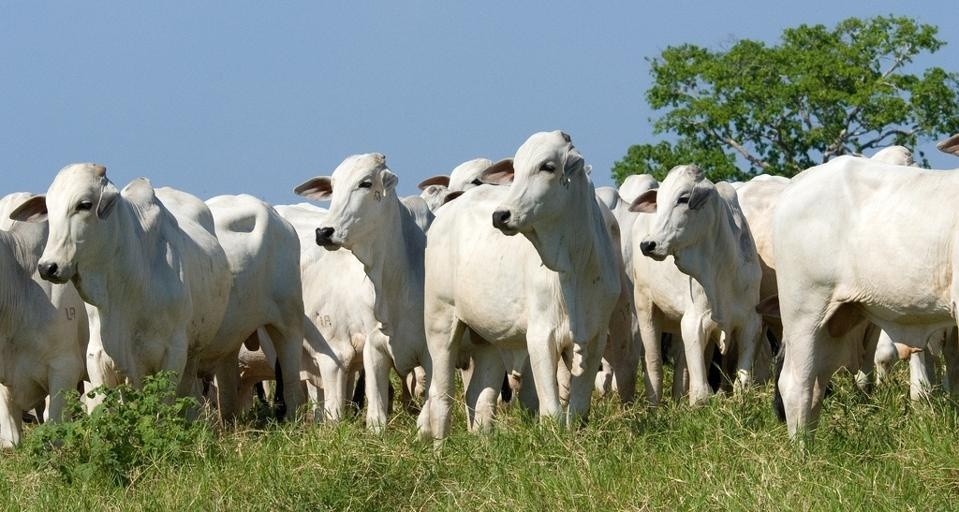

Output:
0,129,958,462
